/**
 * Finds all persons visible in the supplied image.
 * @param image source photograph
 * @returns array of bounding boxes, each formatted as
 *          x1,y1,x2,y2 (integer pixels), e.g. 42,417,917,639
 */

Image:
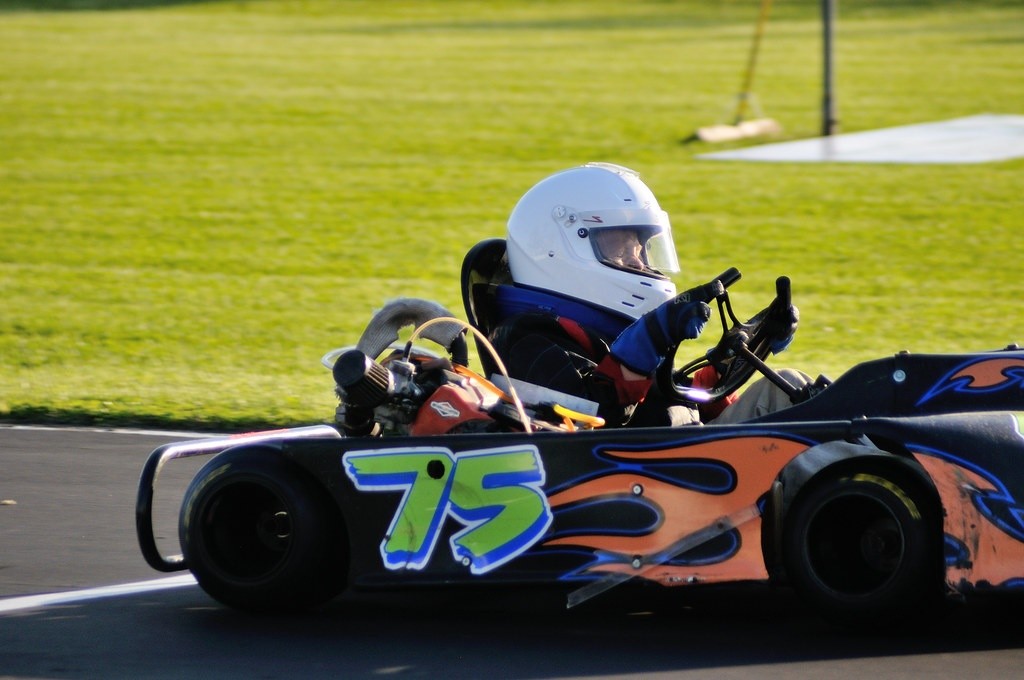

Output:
478,162,815,429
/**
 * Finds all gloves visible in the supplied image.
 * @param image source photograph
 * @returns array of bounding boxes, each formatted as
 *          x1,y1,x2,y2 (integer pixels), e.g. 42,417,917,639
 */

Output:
610,279,726,377
707,304,800,379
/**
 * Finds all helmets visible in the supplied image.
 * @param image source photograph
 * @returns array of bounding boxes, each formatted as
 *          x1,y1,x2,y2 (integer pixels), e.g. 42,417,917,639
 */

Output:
505,162,677,321
